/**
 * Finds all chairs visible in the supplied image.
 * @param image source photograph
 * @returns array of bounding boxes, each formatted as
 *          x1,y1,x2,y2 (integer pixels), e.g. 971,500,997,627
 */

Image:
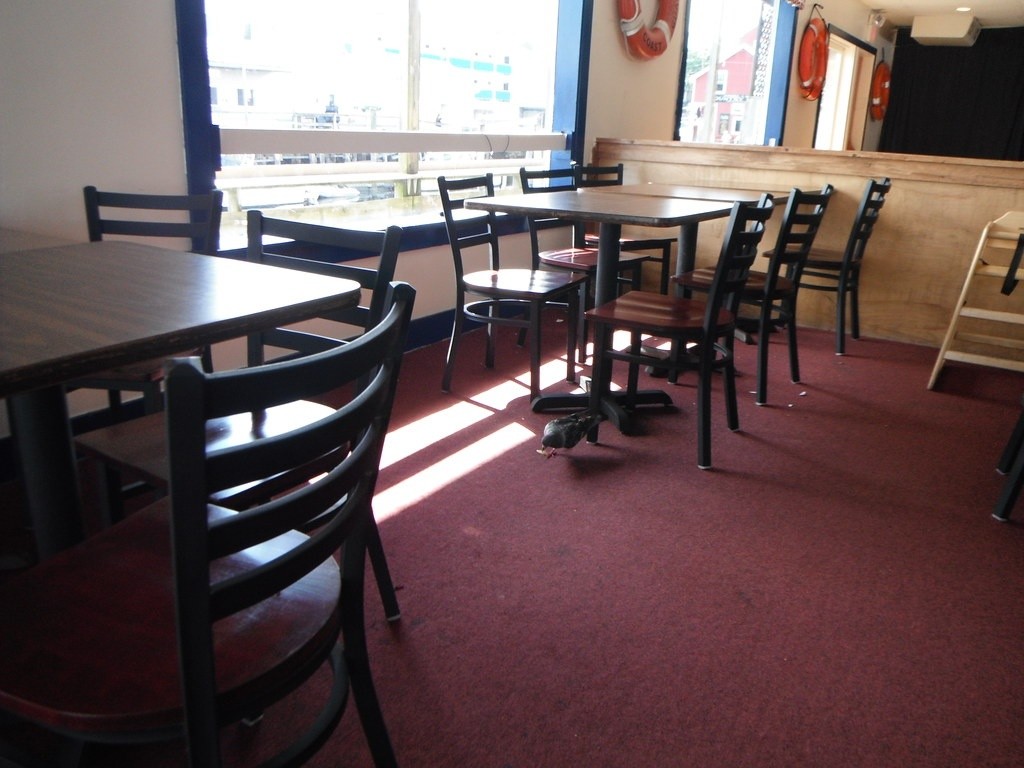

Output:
669,183,834,407
762,178,892,356
584,193,775,469
927,210,1024,390
67,185,223,424
517,168,651,363
438,173,588,411
571,164,678,295
74,211,406,623
0,281,418,768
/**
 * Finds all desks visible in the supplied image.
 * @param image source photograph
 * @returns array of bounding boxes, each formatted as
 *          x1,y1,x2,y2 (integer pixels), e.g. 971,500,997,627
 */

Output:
577,182,793,379
0,241,361,563
647,169,830,345
463,190,737,435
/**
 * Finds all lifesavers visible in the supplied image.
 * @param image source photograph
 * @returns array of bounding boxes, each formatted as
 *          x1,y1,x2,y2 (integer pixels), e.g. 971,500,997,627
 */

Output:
618,0,681,60
799,18,831,101
869,61,891,122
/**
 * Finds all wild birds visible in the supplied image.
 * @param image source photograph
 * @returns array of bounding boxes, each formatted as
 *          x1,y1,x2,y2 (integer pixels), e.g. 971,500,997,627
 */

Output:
542,407,609,459
